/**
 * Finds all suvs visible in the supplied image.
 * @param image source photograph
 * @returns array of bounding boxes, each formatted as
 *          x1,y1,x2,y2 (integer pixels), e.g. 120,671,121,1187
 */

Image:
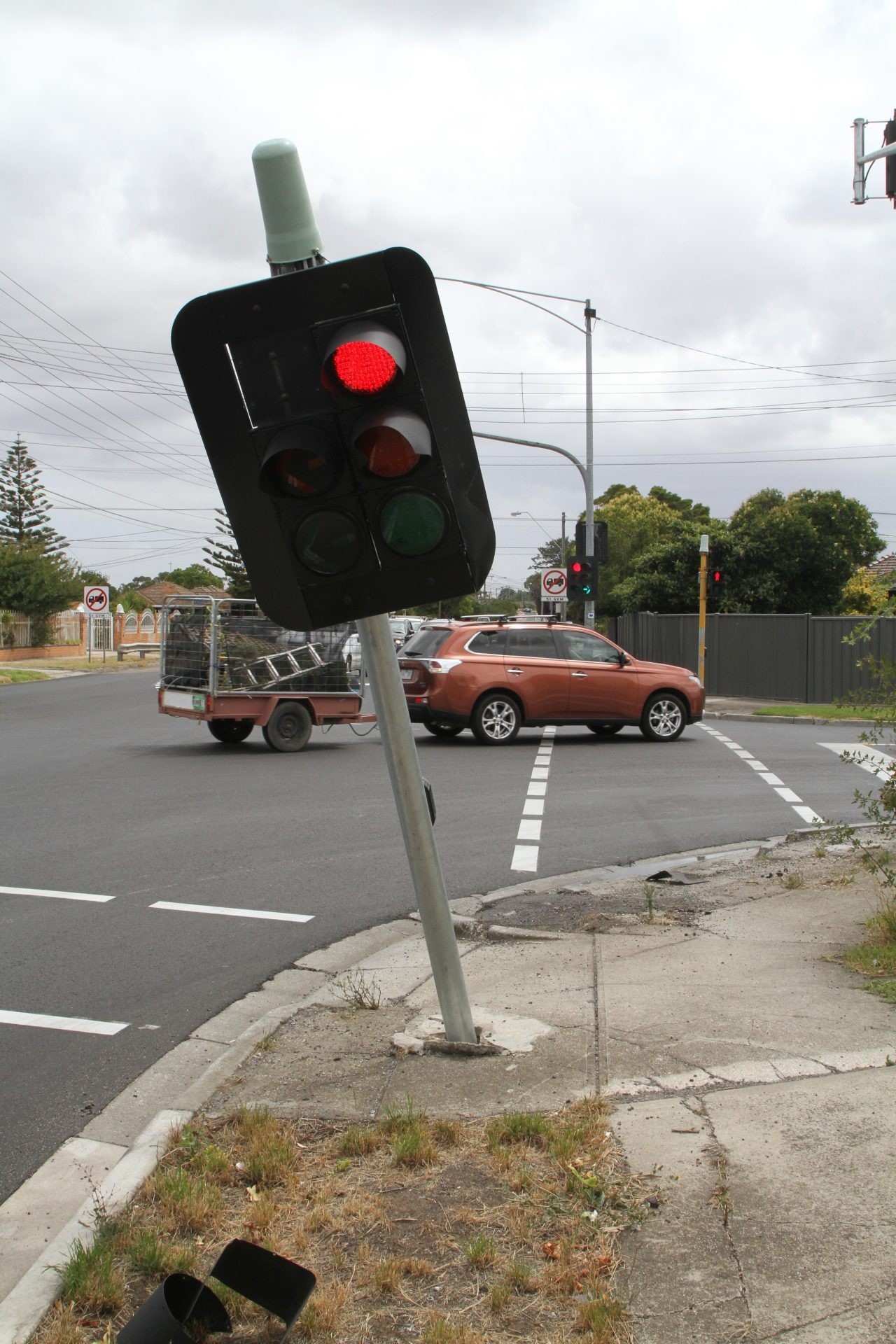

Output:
393,616,707,743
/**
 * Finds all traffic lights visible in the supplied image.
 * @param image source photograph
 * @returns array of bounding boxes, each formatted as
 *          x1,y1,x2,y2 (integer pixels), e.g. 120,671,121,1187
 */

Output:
167,249,499,631
567,556,598,601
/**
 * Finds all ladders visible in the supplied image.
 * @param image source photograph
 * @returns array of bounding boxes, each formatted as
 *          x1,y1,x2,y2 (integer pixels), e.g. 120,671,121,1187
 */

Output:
233,641,335,691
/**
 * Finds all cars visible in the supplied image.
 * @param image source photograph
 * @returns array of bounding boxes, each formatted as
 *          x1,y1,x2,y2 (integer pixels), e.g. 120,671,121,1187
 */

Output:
278,613,543,675
